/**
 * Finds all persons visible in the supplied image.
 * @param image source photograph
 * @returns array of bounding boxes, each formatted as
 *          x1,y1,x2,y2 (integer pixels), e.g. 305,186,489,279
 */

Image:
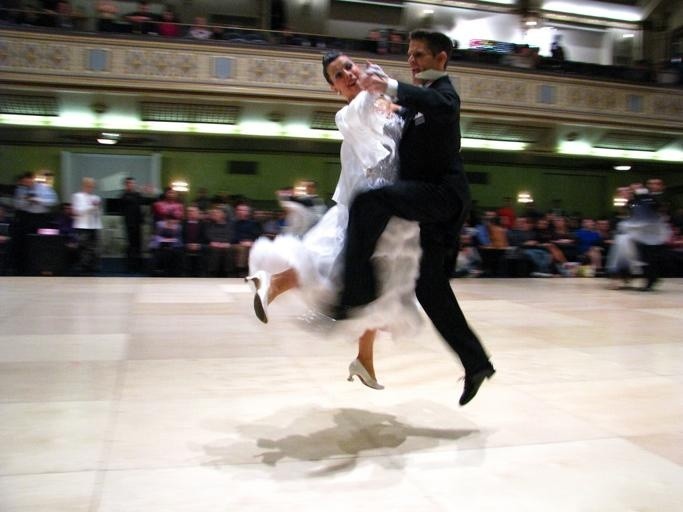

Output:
0,1,566,74
7,172,103,278
119,178,327,280
611,177,675,292
319,29,498,407
241,48,427,392
454,204,612,278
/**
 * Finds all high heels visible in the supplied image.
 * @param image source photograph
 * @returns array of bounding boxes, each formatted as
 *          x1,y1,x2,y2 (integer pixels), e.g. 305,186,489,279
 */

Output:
244,268,270,325
347,358,385,390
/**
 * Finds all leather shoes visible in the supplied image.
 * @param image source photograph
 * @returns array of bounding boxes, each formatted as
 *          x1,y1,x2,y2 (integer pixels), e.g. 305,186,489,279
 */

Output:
327,289,379,320
458,360,496,405
